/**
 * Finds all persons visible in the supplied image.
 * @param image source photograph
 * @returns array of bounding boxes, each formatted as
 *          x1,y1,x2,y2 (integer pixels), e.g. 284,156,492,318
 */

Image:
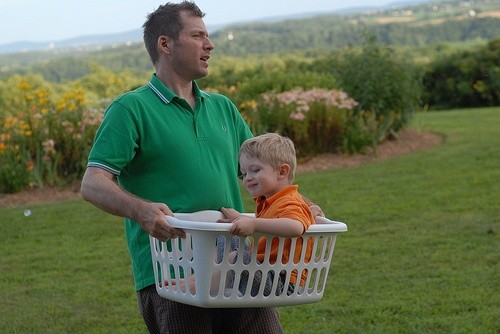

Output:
81,0,325,334
159,132,317,298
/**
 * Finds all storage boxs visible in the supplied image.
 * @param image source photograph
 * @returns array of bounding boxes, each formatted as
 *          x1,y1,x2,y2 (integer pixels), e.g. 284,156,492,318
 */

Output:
149,209,348,308
